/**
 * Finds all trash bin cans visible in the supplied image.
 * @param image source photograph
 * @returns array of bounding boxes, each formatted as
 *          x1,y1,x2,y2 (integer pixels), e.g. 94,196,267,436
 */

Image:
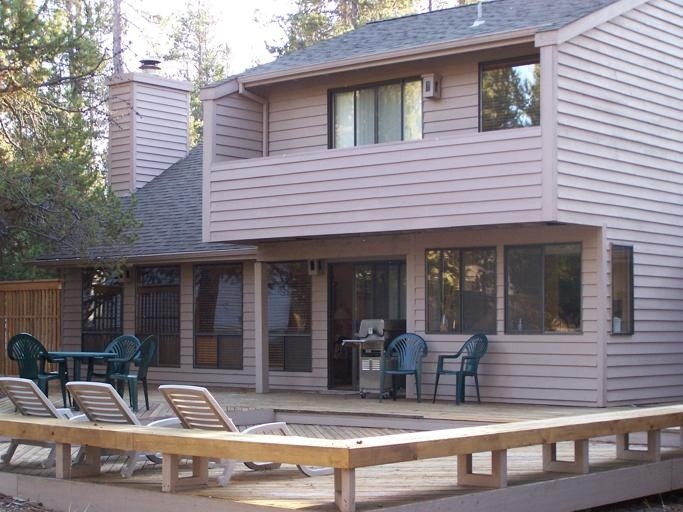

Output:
358,318,405,400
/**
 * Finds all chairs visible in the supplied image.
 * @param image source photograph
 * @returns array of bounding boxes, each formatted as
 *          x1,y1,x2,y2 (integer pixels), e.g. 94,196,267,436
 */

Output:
5,331,156,410
431,332,488,405
0,377,186,477
156,385,333,488
378,333,428,403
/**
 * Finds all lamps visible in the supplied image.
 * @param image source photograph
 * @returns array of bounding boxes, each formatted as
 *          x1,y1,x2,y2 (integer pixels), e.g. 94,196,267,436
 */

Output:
306,256,323,276
421,73,443,100
